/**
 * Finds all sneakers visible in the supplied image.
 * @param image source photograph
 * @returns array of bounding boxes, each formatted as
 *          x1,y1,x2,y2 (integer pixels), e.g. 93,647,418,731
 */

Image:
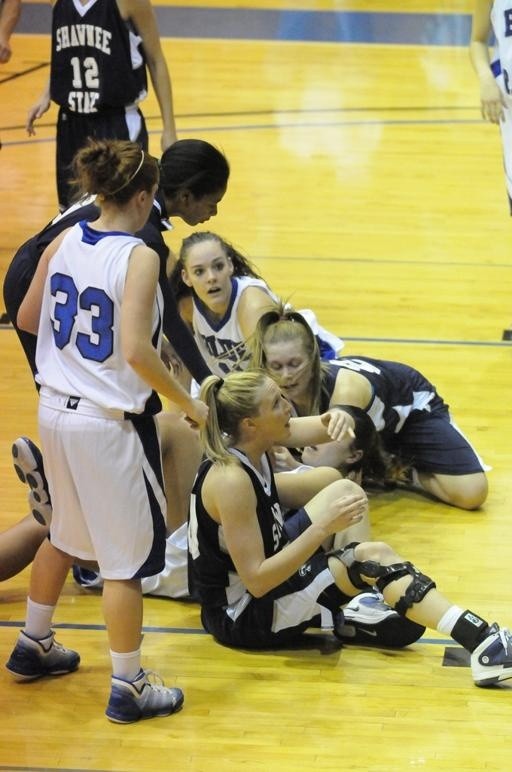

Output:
5,629,81,684
470,621,512,687
11,435,57,526
333,591,426,648
105,669,185,723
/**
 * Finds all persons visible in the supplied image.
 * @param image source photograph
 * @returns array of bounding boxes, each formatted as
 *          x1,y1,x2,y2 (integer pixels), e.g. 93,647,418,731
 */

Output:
2,137,211,732
1,136,512,687
0,0,23,69
467,0,511,347
24,0,180,220
